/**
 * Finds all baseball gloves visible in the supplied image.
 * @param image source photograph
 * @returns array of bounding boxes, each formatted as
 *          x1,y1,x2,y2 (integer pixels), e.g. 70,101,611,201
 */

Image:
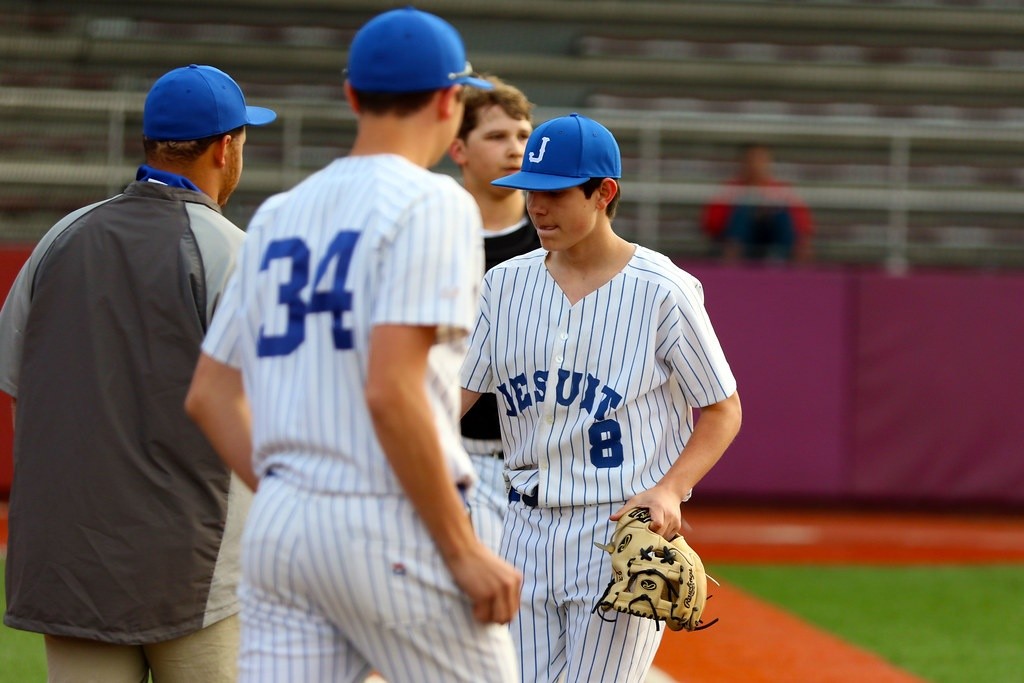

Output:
589,507,722,633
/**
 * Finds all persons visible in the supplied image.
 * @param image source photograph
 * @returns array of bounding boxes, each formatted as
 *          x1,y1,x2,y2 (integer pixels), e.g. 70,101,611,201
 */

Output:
0,61,279,679
443,73,544,448
452,114,745,683
697,143,818,263
180,9,527,682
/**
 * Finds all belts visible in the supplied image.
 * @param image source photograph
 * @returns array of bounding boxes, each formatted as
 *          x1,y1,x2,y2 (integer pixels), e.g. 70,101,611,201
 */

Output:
509,487,538,507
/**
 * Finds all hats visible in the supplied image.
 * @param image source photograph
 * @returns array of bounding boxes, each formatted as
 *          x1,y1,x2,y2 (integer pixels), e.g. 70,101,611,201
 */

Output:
346,6,494,94
490,112,622,191
143,64,277,141
